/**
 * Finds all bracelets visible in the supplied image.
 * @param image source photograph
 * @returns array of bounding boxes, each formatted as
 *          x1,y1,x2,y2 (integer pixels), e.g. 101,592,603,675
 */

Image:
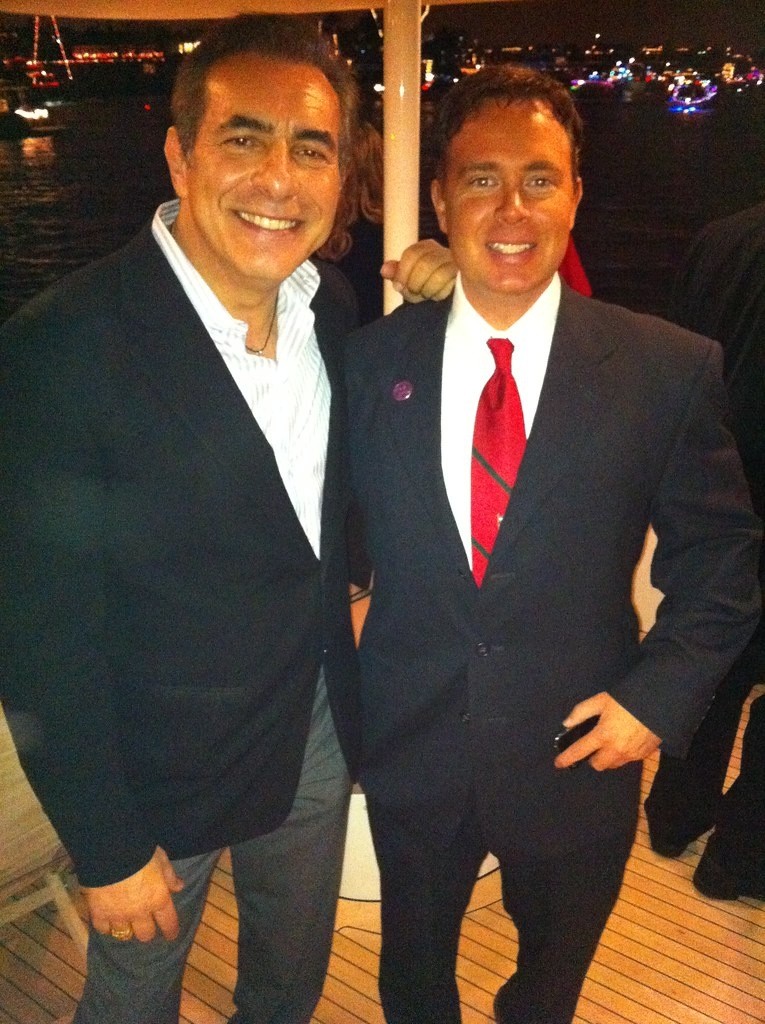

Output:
350,589,371,603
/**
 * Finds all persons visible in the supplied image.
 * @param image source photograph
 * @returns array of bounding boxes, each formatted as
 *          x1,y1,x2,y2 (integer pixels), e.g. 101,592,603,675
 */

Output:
644,205,765,900
322,66,765,1024
0,11,461,1024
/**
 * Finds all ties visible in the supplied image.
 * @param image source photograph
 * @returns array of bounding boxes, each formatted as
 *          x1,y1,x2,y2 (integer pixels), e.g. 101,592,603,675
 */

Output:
471,337,525,591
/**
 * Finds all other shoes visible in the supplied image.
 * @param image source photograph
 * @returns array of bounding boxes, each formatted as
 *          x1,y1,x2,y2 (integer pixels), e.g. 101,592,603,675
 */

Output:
643,790,726,859
691,847,765,901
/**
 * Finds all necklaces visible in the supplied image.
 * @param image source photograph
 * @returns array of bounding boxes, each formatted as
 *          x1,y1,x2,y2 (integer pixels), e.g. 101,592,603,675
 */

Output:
246,290,278,354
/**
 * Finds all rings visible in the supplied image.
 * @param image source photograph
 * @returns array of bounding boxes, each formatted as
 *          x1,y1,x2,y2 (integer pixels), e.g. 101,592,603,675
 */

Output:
111,930,133,941
